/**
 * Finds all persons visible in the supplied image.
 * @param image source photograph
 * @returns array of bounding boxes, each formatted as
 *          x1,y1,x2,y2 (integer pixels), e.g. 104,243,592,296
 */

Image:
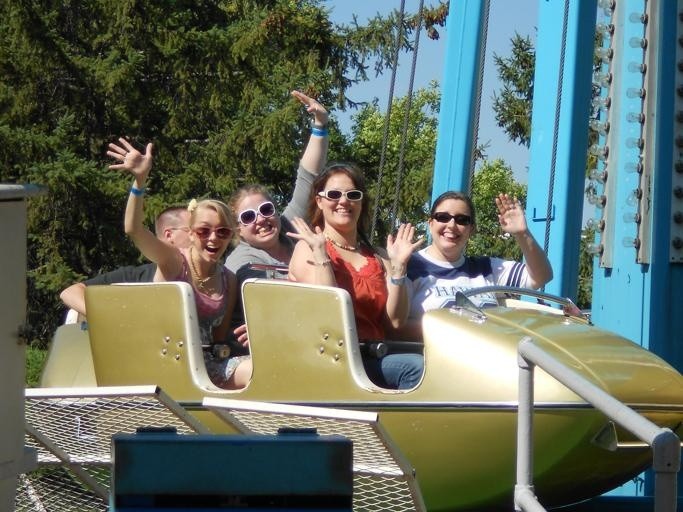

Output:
403,191,554,344
224,90,330,353
286,161,426,391
106,137,253,389
60,204,191,316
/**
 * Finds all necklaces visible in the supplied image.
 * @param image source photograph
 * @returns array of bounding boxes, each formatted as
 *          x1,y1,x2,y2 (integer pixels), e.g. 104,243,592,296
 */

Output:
188,246,218,291
325,232,363,251
265,248,290,260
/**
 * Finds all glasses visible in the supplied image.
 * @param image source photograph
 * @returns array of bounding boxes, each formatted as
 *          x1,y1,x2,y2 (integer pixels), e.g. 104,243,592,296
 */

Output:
431,212,475,225
194,226,233,240
318,189,364,202
237,200,276,226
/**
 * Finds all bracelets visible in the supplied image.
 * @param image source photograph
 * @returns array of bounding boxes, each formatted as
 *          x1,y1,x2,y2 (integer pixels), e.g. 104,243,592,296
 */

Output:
313,258,332,267
310,127,328,137
130,186,146,197
390,273,408,286
312,121,327,130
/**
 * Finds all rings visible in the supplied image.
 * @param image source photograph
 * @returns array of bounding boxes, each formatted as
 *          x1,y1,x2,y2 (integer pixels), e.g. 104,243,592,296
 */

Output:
302,228,307,232
